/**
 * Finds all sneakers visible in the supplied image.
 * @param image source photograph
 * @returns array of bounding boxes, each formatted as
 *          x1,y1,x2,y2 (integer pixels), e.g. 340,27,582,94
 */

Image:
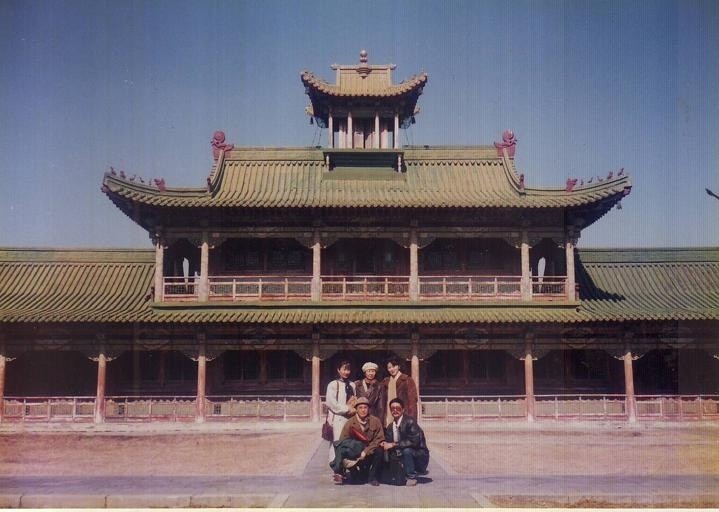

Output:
406,478,417,487
370,480,379,486
333,474,344,485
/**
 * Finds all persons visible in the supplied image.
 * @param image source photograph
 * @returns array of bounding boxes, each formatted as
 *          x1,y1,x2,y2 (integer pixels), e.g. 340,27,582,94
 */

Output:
337,396,387,487
378,353,419,434
325,358,356,482
379,398,430,487
354,361,385,422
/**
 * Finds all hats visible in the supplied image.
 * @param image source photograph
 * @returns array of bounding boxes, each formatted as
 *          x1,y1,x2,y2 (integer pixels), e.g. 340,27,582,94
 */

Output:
353,397,371,409
362,362,379,373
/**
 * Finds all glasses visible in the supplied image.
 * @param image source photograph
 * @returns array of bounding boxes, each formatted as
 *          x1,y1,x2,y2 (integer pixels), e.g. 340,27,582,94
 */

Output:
391,406,403,412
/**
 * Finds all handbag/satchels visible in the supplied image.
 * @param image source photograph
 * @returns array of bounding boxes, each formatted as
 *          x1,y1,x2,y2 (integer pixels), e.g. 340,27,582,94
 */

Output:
321,421,334,442
